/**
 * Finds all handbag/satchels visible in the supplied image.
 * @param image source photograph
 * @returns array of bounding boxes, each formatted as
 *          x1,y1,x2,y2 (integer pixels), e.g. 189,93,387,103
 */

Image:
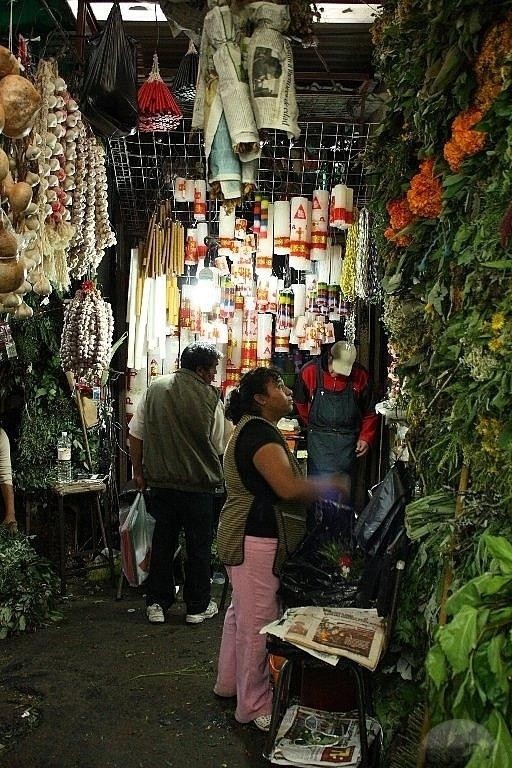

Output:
117,482,156,588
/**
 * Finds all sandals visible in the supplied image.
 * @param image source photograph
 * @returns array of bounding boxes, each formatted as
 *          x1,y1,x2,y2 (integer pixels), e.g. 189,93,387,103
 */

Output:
254,715,272,731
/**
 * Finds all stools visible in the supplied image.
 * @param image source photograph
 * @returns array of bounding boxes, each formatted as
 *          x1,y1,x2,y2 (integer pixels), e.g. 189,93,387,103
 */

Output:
260,633,377,768
50,478,117,598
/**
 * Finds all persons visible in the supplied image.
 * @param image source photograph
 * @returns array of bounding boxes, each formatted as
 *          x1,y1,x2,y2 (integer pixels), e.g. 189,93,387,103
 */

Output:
125,342,234,626
0,430,19,533
252,48,283,84
212,366,351,733
292,340,381,533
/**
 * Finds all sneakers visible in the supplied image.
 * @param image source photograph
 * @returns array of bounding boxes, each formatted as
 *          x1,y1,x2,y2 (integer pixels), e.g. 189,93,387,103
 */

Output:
147,603,165,623
184,601,218,623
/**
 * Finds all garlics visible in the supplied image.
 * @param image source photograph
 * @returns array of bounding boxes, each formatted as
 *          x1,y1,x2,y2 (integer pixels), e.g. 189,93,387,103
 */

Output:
0,77,114,379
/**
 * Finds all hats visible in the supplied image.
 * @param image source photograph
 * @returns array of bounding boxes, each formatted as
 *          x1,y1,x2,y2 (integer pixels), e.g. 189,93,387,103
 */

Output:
331,340,358,376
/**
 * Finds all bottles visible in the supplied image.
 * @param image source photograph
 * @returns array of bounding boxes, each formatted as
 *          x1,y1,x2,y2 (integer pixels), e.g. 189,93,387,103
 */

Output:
57,431,73,484
